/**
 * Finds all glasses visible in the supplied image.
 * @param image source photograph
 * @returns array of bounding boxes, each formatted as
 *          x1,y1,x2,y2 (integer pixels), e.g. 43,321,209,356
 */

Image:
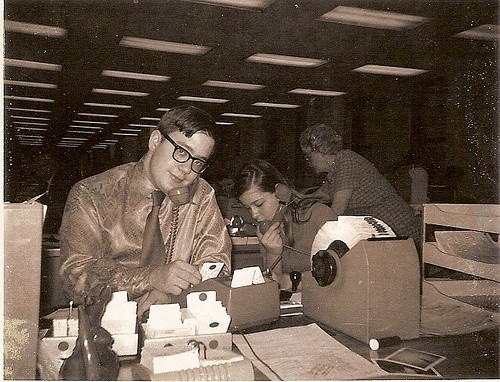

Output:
163,134,210,174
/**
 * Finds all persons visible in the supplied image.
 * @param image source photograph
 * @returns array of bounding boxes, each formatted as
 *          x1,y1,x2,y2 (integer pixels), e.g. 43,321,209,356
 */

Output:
59,105,234,313
231,156,339,293
434,155,498,204
385,149,433,209
292,123,436,256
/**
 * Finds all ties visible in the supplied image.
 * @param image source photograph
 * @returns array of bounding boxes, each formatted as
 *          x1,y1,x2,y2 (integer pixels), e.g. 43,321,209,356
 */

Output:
139,191,166,268
